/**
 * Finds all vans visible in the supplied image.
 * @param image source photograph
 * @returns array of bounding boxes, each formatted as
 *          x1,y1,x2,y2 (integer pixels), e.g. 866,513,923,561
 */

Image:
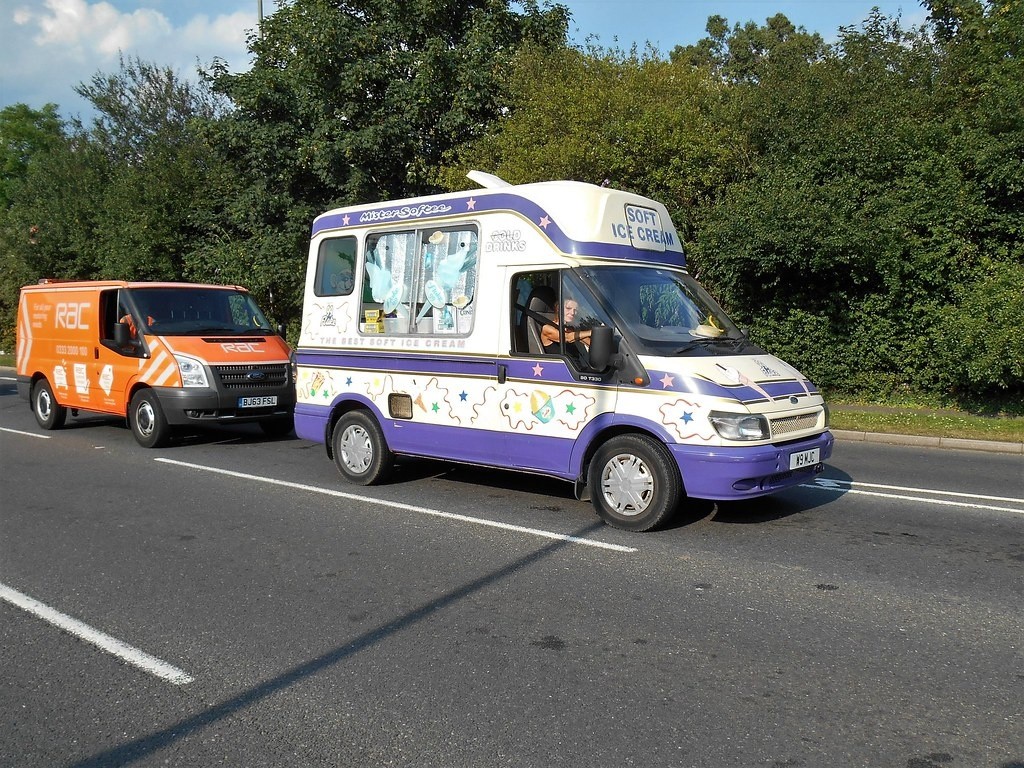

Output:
14,277,298,447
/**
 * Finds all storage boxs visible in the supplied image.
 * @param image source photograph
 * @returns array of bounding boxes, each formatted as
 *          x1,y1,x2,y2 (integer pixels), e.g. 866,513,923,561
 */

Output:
364,309,433,334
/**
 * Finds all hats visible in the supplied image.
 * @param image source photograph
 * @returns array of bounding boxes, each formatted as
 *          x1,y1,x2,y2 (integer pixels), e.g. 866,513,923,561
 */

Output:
688,325,720,340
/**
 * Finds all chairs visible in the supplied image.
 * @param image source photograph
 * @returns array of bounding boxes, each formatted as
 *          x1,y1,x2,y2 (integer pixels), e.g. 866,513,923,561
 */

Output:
522,283,646,353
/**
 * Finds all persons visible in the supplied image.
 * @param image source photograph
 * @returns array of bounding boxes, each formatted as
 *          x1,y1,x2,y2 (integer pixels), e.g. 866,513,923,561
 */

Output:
540,295,592,370
119,313,156,339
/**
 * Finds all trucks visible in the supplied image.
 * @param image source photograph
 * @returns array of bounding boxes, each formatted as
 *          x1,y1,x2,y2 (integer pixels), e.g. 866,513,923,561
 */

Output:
291,170,833,532
